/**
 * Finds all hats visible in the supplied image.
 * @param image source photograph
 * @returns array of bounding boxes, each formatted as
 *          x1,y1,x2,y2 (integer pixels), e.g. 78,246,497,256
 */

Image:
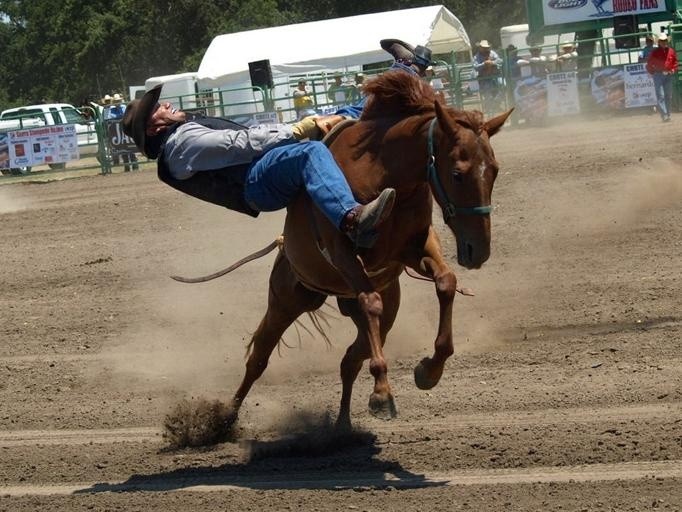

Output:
100,94,113,105
475,39,494,49
117,82,166,160
505,44,517,52
559,41,575,49
111,94,124,103
653,33,672,46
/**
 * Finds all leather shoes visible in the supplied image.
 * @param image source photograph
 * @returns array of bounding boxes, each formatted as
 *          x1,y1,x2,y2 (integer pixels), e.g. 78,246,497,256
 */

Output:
342,186,398,250
663,114,672,123
378,36,436,74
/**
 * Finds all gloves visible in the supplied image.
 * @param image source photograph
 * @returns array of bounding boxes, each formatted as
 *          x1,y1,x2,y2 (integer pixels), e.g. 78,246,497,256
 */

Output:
291,114,325,142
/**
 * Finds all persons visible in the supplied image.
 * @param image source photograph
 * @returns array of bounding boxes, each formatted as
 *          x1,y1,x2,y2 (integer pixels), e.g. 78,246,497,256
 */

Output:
528,45,546,73
110,92,140,172
351,72,370,106
635,34,659,65
117,35,434,249
326,70,354,108
556,40,580,72
645,31,680,122
471,38,504,114
292,76,315,118
502,43,524,80
99,92,124,166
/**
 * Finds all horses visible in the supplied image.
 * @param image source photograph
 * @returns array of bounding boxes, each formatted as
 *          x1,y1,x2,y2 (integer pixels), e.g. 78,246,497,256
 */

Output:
219,68,515,442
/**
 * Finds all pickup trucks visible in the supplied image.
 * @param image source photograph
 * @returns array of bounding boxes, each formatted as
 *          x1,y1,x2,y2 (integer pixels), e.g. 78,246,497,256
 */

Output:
0,103,120,176
